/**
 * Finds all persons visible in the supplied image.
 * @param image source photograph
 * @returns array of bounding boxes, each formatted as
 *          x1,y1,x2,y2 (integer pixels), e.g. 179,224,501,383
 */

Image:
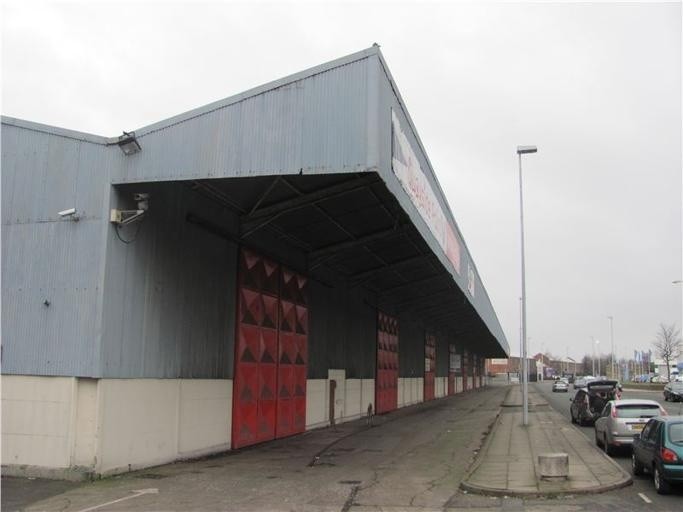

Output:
614,385,622,400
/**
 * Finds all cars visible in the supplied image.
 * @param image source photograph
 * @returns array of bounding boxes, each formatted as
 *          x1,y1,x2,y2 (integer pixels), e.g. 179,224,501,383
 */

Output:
663,381,683,402
631,415,683,494
552,375,596,392
631,375,669,383
570,380,623,427
594,399,668,455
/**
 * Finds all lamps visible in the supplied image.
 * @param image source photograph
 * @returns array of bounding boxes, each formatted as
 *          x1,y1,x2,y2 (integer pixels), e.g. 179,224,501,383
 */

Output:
116,131,141,156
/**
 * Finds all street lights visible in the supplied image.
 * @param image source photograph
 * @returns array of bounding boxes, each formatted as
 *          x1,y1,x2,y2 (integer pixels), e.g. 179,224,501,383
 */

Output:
517,146,537,425
608,316,614,378
